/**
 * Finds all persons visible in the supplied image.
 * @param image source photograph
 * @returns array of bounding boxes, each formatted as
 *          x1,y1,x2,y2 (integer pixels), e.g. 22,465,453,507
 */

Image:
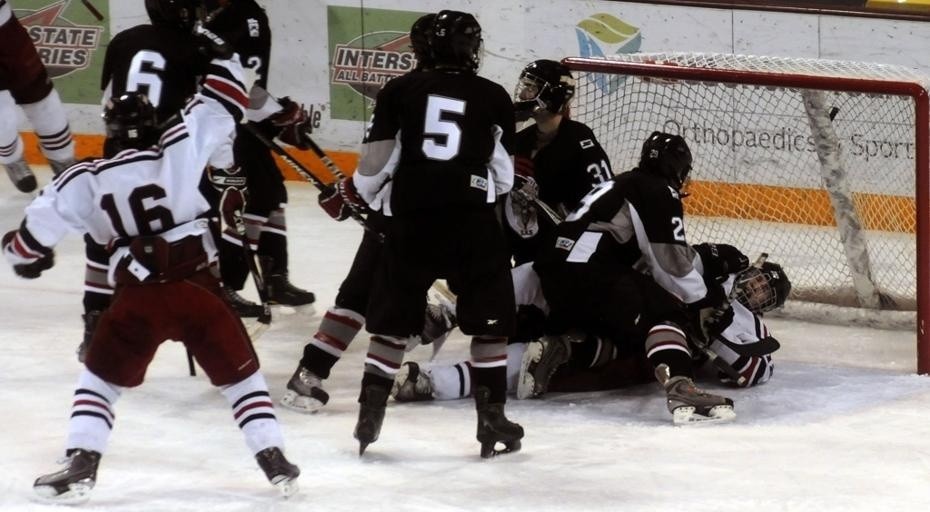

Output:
1,1,318,497
279,9,791,458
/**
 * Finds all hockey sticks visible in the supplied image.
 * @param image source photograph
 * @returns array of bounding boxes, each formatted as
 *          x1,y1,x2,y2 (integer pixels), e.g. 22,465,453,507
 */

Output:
535,199,779,357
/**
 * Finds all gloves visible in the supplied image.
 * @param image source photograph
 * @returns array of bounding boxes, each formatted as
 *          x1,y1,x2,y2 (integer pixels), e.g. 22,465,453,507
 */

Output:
259,95,319,153
510,173,543,212
687,289,738,350
1,226,60,282
315,173,372,225
691,239,751,285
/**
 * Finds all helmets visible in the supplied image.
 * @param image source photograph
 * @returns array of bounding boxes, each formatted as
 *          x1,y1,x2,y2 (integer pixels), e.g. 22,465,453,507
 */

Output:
143,0,200,25
431,9,483,53
753,258,793,313
639,129,695,190
101,87,160,142
410,13,439,57
519,58,578,117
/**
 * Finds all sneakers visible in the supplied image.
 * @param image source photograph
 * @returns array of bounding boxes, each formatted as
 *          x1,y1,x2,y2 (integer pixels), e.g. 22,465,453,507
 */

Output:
1,150,40,193
47,159,75,178
32,447,102,495
220,284,265,318
261,272,316,307
254,445,302,483
285,360,331,406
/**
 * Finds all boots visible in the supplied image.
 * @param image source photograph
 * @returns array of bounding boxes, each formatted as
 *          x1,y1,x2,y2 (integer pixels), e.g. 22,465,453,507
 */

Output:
469,386,526,443
650,364,739,414
349,383,388,442
418,301,458,345
393,360,436,403
523,328,584,401
